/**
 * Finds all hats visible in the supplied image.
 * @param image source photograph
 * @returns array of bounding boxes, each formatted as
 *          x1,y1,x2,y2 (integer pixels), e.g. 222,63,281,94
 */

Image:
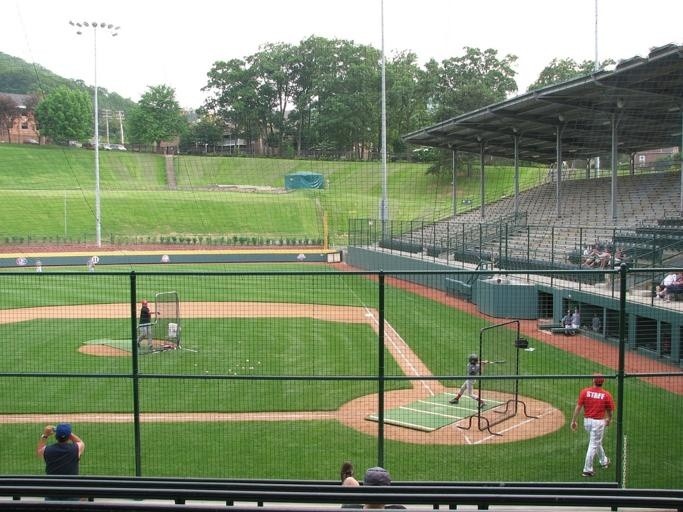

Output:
141,300,147,304
363,466,391,485
592,371,604,384
56,422,72,437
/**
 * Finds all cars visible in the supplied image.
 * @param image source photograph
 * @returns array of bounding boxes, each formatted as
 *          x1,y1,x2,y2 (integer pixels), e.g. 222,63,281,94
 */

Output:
24,139,38,144
69,139,126,151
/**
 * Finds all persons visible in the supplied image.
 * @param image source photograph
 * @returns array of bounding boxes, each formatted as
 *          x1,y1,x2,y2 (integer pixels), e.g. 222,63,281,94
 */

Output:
137,299,160,350
448,353,494,408
656,272,683,303
654,273,680,300
497,279,502,285
570,371,615,477
87,256,95,271
35,257,42,272
37,423,86,501
561,309,572,335
581,244,632,269
590,310,602,331
572,307,581,328
340,460,408,509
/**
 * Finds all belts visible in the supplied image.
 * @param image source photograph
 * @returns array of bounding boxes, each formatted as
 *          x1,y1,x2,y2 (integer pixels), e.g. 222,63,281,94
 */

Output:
585,416,603,420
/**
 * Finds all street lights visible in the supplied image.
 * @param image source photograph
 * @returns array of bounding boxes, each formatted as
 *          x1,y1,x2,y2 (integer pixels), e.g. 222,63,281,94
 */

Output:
70,20,121,247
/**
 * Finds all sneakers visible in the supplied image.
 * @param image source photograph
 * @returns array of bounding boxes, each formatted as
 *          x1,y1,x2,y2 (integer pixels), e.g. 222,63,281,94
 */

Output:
449,398,459,404
601,456,610,470
137,341,141,348
477,400,487,408
149,346,156,352
582,470,596,477
654,292,670,302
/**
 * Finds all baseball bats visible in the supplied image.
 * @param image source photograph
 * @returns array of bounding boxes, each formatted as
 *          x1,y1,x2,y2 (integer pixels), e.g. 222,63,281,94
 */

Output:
485,360,504,364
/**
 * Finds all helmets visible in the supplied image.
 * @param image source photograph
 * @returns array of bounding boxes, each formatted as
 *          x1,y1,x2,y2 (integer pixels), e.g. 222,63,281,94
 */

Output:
468,354,478,364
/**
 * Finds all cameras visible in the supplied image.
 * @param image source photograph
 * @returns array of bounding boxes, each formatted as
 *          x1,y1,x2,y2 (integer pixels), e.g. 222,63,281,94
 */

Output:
52,427,56,432
